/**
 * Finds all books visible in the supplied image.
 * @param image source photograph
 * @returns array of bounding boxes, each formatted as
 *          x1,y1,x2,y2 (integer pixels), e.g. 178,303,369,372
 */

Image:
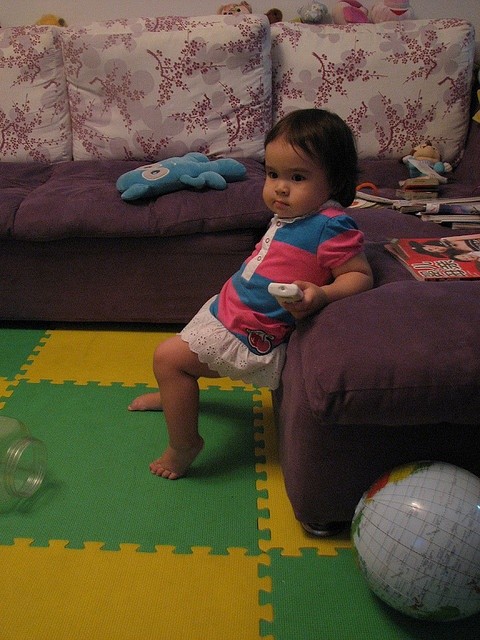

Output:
350,186,480,230
384,234,480,283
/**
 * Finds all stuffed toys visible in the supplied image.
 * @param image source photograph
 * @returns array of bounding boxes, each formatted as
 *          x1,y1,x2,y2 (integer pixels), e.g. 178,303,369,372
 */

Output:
366,0,412,23
293,2,330,24
114,152,247,202
331,0,367,26
402,140,454,179
219,0,282,23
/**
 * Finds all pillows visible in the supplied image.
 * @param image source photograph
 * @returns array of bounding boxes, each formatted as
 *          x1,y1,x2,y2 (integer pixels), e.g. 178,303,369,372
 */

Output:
60,15,273,163
268,17,475,173
0,24,73,164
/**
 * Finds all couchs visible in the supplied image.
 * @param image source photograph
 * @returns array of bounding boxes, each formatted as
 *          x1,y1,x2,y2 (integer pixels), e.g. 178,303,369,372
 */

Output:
0,14,480,538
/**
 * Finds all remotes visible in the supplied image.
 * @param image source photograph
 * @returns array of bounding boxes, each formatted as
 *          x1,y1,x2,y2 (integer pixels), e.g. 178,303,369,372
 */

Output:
266,281,305,304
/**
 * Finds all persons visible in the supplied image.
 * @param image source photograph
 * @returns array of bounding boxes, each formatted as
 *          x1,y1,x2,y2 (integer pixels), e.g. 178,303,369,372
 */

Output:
409,233,480,252
451,252,480,273
125,111,375,483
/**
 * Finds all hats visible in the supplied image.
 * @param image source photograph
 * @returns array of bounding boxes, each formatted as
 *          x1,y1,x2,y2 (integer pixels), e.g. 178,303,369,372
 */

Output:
409,241,446,258
